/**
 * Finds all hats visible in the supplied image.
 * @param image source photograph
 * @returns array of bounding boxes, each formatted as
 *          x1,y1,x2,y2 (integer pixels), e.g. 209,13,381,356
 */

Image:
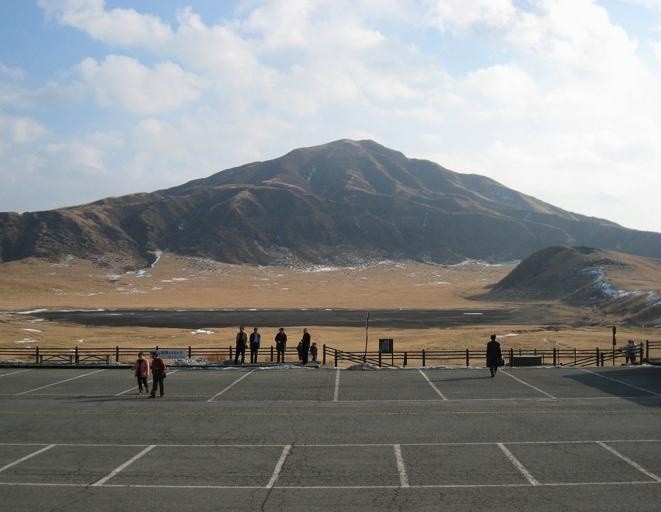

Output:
278,328,283,330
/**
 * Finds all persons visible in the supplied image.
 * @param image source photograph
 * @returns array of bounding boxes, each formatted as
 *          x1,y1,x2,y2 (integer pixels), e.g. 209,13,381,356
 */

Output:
631,340,637,361
232,326,248,366
296,342,302,361
621,339,632,364
309,341,319,362
131,350,150,395
486,333,503,377
301,328,311,364
145,352,166,398
275,327,288,363
250,327,262,364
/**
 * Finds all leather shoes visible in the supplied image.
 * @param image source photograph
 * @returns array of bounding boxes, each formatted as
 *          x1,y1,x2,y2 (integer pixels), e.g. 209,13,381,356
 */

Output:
148,395,154,398
